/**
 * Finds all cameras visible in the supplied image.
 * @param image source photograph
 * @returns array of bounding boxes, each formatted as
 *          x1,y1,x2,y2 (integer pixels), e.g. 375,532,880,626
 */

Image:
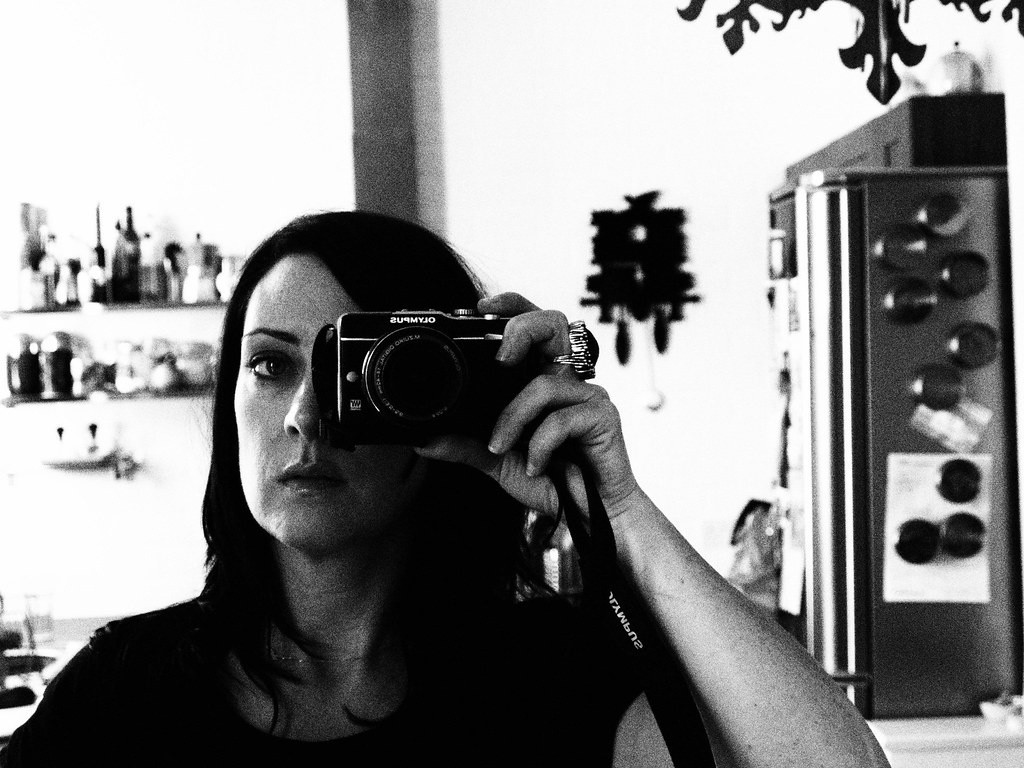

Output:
338,308,540,453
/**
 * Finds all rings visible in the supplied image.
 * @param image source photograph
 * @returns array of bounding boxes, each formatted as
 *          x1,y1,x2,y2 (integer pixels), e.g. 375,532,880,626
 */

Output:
551,323,603,379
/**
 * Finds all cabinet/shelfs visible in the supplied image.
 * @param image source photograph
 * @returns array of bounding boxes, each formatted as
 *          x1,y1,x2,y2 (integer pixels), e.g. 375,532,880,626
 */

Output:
2,291,239,409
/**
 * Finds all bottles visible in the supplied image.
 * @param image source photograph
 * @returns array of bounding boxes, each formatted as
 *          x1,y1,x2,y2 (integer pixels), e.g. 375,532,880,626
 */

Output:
112,207,138,302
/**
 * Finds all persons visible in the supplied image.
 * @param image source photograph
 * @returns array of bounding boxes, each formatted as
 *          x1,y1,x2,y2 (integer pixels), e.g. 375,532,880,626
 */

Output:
0,209,892,768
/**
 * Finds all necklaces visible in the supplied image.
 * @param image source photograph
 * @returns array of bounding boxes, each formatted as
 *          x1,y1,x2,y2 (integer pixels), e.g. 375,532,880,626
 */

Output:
264,623,371,677
